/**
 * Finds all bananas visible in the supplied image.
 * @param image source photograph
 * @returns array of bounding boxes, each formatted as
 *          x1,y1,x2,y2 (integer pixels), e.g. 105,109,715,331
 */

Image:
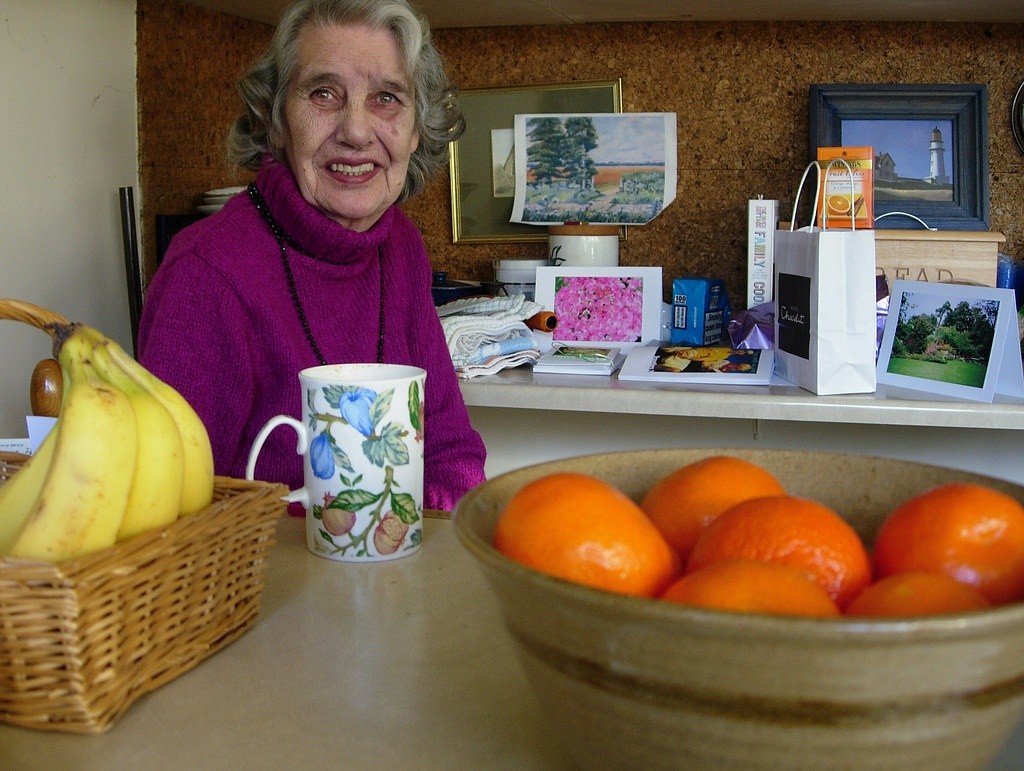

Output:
1,319,216,561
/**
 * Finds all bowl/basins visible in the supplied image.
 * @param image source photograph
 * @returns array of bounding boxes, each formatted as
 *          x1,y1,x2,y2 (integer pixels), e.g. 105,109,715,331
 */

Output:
451,451,1024,771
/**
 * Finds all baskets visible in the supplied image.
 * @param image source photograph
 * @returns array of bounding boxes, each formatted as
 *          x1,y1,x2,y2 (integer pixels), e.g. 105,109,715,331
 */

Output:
0,298,291,736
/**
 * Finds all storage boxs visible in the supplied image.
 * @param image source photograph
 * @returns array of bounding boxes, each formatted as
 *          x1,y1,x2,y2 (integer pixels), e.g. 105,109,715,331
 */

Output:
816,145,874,229
669,277,726,345
745,198,780,309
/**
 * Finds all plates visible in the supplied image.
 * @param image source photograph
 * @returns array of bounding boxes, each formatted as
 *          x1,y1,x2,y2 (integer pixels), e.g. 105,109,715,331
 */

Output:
197,188,248,215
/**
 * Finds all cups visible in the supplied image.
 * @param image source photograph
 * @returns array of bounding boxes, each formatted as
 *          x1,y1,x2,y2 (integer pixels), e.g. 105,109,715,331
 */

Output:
246,363,427,561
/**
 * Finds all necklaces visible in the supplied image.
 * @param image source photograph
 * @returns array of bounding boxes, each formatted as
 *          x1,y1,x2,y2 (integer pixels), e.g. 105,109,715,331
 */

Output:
246,180,385,367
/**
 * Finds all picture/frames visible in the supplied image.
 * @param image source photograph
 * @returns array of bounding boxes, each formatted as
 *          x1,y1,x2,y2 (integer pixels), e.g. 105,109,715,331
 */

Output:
448,77,628,247
807,84,990,232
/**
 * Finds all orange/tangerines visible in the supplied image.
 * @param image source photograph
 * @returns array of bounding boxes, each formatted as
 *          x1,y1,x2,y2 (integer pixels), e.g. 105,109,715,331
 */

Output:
490,454,1024,621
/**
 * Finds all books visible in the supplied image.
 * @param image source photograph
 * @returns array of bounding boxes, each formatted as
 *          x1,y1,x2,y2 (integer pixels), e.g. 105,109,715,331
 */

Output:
617,346,776,387
533,345,627,376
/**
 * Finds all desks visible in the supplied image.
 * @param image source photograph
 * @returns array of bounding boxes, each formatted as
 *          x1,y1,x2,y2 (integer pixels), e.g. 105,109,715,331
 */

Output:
0,512,1024,771
455,370,1024,506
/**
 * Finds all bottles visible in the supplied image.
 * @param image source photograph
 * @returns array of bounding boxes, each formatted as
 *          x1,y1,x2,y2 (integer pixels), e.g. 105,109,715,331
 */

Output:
491,257,549,302
548,224,619,267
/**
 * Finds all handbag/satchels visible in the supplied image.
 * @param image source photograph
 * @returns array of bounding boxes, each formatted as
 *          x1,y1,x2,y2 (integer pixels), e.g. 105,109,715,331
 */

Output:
773,158,877,396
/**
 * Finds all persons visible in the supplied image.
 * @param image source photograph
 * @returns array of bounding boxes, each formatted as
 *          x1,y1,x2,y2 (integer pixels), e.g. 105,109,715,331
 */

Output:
136,0,487,515
653,346,754,374
